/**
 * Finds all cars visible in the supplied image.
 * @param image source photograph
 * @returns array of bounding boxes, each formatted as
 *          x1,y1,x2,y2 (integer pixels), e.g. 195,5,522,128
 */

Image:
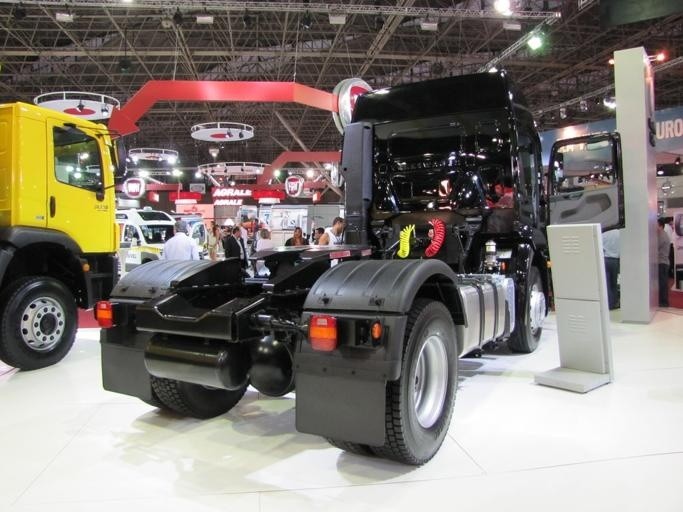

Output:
111,207,207,275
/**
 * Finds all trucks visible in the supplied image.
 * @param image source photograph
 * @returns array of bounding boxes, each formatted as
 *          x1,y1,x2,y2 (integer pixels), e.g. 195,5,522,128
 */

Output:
95,69,626,466
1,99,123,371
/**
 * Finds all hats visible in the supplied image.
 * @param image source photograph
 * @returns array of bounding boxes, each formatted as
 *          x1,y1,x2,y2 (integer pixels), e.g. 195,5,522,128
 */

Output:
175,221,188,233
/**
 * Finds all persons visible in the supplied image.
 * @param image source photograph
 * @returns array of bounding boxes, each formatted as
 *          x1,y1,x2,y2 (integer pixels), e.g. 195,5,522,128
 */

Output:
602,230,620,310
490,177,514,208
657,217,673,307
284,217,344,246
164,222,199,260
208,218,259,269
